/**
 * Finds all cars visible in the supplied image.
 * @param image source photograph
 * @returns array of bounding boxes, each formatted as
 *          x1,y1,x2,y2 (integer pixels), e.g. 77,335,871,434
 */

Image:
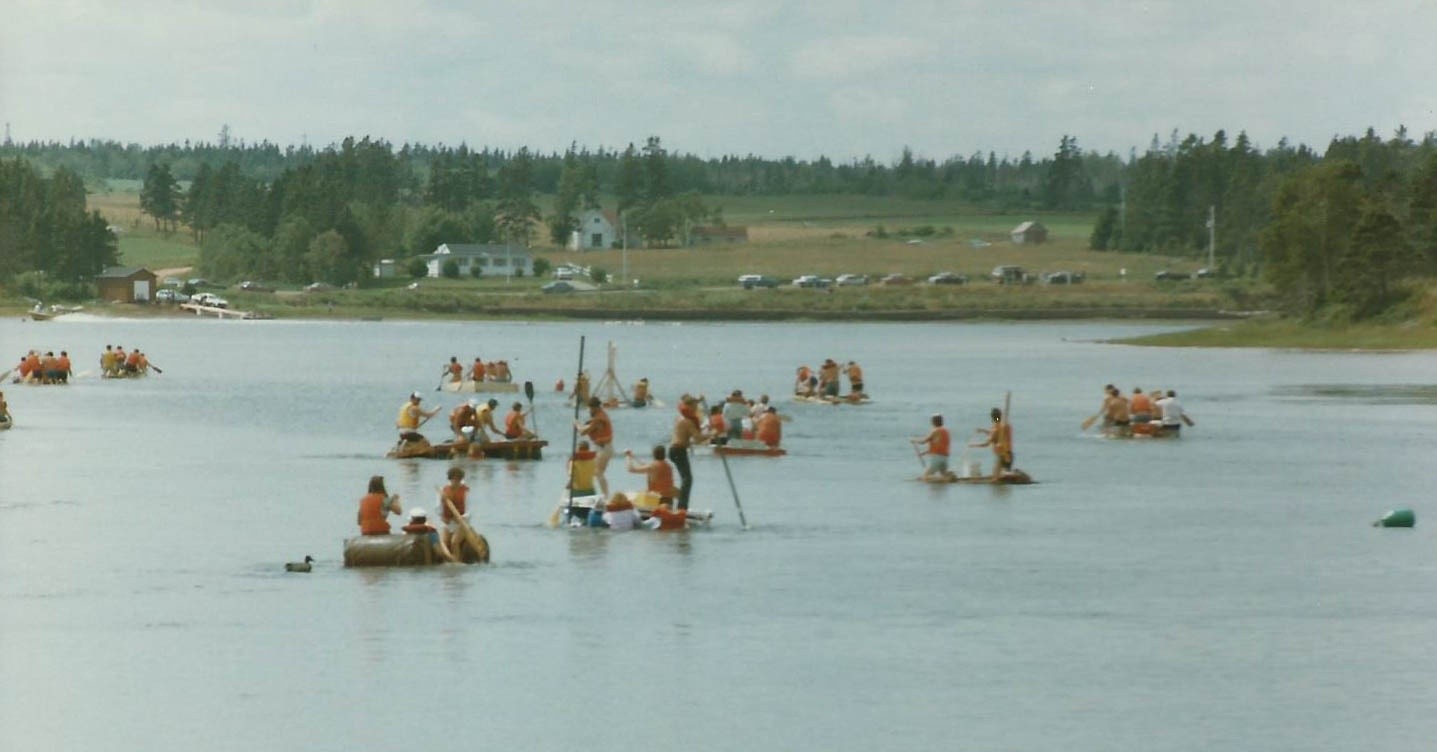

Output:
154,289,174,302
541,281,574,293
164,277,178,286
304,283,328,292
186,278,205,286
1047,272,1081,284
1155,271,1190,281
105,224,124,233
170,291,190,304
836,274,864,286
1195,266,1219,280
794,275,831,287
241,281,263,290
556,264,583,280
190,293,230,308
930,273,968,286
882,273,912,284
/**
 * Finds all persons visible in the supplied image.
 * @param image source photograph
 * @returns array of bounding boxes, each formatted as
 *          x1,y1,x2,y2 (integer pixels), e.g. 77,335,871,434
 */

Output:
0,392,13,422
701,389,782,446
357,475,400,535
630,377,653,408
1102,384,1194,431
565,440,596,497
669,394,719,511
436,467,471,560
968,408,1014,478
406,509,445,562
17,350,73,384
584,492,689,530
100,344,148,377
394,392,536,446
625,445,674,506
443,357,512,382
573,397,614,497
574,372,591,405
795,358,863,399
911,414,951,479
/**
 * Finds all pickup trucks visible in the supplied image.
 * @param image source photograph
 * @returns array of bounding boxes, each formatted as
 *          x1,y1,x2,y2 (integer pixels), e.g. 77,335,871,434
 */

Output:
738,274,780,289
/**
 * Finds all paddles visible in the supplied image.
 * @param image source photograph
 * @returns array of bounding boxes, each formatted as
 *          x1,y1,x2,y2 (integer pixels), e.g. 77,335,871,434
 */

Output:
629,451,682,499
1082,409,1104,431
416,405,443,429
434,364,447,392
524,379,542,462
698,392,748,528
146,363,162,373
1151,391,1195,428
991,390,1012,484
912,438,926,468
0,368,13,382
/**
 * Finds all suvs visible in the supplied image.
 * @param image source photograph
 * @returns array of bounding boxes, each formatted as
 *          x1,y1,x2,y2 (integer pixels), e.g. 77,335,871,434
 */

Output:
992,264,1036,283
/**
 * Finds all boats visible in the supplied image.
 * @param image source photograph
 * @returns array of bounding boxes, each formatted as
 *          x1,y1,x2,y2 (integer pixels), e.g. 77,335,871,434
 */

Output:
29,310,53,320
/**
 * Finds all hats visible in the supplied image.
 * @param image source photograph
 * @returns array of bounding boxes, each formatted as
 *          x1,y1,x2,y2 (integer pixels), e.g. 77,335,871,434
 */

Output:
412,391,422,398
